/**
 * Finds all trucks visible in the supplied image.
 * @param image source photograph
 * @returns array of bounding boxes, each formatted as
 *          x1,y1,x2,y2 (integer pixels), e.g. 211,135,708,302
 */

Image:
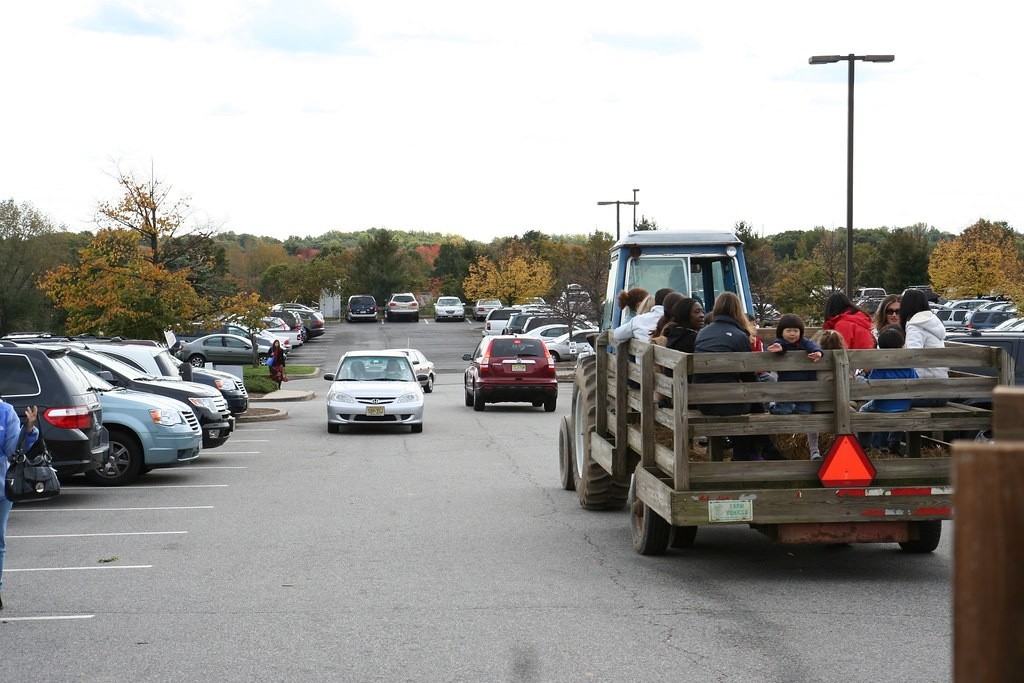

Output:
557,228,1020,557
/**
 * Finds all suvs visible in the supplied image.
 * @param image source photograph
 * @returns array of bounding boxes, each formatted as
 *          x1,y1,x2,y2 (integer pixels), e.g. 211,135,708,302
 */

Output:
461,335,559,412
0,332,251,488
230,302,326,344
807,285,1024,452
384,292,419,323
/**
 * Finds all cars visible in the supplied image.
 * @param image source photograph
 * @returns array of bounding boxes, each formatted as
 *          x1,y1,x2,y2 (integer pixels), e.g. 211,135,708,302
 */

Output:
472,284,602,363
433,296,466,322
750,303,781,326
174,323,287,366
323,348,436,432
346,295,378,322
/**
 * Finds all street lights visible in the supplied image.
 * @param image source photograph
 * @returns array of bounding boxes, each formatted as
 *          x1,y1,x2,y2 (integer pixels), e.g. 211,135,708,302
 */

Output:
809,53,894,302
633,188,639,231
596,202,639,242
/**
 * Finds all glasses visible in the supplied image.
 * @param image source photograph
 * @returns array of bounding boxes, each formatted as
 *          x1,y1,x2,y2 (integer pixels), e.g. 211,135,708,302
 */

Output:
886,307,903,315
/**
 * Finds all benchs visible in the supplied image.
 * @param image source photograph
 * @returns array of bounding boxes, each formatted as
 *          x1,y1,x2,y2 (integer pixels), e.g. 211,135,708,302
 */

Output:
607,378,973,461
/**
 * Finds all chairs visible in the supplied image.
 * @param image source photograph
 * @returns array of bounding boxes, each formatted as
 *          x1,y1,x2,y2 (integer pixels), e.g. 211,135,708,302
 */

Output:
521,347,537,354
350,361,366,378
494,345,514,357
385,363,402,379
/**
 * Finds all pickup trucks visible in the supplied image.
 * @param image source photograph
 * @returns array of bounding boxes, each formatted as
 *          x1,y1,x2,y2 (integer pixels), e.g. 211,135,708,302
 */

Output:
263,329,303,348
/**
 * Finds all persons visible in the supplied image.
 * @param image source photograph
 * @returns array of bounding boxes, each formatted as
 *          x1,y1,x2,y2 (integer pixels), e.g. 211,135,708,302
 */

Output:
871,289,949,448
661,298,705,410
744,313,778,382
822,292,874,349
381,360,398,377
267,340,286,390
871,294,903,349
807,330,846,460
617,287,648,388
614,289,673,366
0,398,39,610
858,324,919,452
649,292,685,400
766,314,824,414
693,291,766,461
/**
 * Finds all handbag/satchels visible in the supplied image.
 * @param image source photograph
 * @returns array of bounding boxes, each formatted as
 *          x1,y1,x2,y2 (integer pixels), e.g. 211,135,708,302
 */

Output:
284,375,290,383
4,413,61,504
266,347,274,366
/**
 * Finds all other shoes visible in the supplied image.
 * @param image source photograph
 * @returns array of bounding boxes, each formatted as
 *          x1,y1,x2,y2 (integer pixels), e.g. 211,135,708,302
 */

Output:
809,450,822,460
698,439,710,445
